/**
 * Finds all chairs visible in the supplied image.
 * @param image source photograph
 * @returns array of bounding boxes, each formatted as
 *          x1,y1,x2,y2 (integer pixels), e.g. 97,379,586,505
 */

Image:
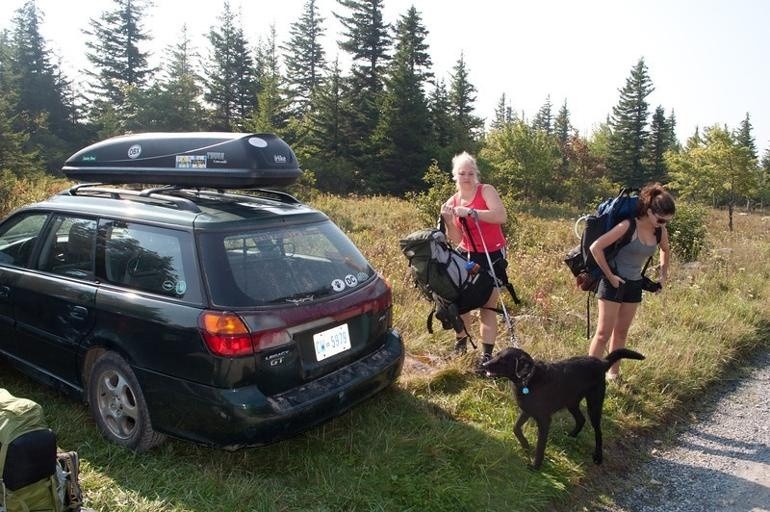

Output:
51,223,92,272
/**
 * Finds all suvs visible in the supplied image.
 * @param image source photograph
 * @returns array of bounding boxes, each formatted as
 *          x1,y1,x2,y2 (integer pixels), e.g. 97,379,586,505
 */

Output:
0,133,406,451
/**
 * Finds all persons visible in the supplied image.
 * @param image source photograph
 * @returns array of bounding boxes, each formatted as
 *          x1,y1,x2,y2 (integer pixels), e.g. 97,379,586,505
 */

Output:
588,180,677,394
439,149,511,375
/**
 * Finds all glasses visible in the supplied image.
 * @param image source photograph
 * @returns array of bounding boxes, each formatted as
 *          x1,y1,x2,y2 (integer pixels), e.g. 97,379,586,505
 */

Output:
652,212,671,224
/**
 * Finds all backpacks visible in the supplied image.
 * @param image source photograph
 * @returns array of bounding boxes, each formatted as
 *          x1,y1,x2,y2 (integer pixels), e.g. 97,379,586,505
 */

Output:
398,208,494,314
564,187,662,291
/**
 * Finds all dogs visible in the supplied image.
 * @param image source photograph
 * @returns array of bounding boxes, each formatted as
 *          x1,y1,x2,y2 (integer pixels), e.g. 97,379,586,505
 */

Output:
479,345,647,474
